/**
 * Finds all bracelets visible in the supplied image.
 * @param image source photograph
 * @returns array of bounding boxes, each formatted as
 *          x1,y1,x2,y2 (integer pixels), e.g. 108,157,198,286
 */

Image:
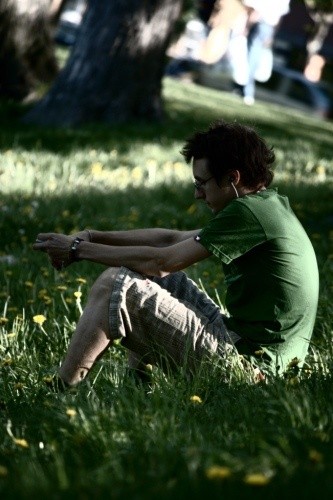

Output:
70,237,83,263
85,229,92,240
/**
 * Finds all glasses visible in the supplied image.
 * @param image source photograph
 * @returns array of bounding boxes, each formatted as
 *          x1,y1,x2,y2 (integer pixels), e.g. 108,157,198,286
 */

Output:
191,173,215,189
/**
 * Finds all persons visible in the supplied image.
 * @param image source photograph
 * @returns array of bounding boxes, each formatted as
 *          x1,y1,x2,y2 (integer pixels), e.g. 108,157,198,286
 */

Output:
159,0,333,105
31,123,321,388
